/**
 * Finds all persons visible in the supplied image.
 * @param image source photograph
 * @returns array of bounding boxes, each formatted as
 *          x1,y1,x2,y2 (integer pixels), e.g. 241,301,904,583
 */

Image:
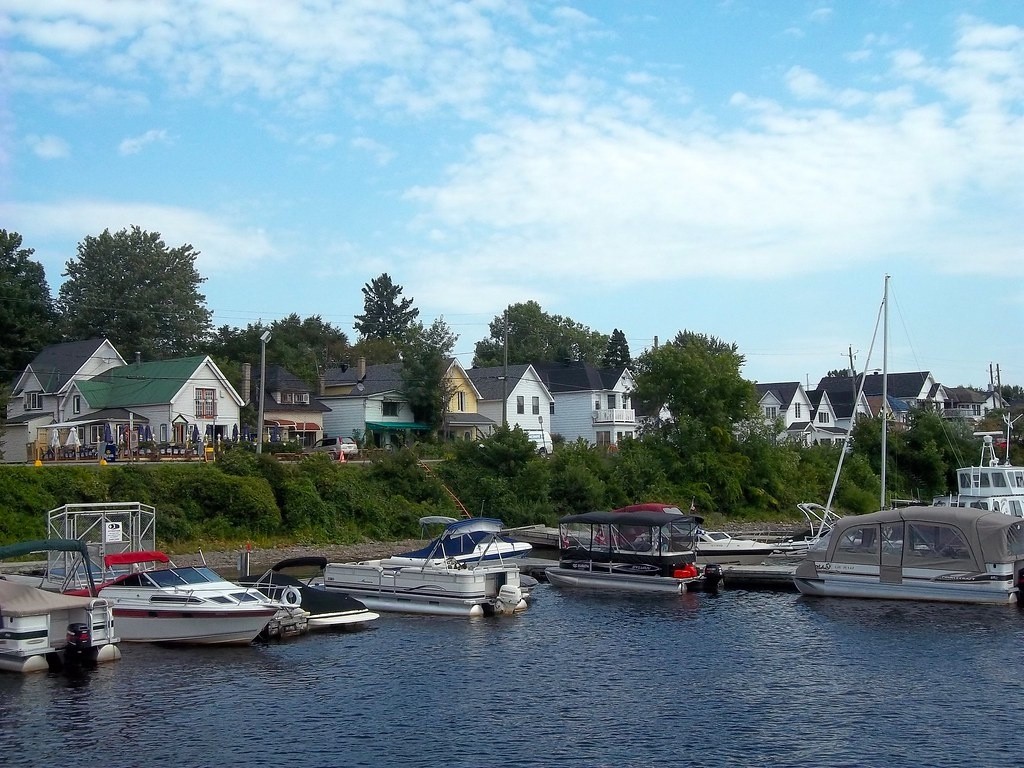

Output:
118,445,128,458
42,445,97,460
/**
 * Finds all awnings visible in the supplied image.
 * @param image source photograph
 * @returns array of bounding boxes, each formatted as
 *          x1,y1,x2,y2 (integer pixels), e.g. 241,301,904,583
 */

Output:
366,421,433,431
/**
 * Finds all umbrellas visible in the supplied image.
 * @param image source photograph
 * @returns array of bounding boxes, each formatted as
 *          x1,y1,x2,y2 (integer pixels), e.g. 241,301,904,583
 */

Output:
138,424,153,442
205,424,211,435
232,423,250,442
52,428,60,447
271,423,280,442
104,423,111,443
65,427,82,446
192,424,199,444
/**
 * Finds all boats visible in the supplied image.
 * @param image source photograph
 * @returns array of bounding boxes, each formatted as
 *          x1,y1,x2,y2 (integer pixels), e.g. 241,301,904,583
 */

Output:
233,556,381,634
544,511,725,597
299,515,534,617
661,530,776,565
0,538,123,676
65,552,280,650
794,505,1024,606
927,430,1024,518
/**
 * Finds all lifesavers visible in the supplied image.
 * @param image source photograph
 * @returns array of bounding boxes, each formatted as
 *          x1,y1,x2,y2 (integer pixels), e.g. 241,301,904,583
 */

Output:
282,585,303,606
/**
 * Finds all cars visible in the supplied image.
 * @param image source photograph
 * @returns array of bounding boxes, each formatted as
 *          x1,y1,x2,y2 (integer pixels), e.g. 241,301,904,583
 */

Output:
310,436,359,461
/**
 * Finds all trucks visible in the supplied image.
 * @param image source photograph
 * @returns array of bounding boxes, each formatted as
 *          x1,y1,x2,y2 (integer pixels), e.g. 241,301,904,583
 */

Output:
520,430,554,458
